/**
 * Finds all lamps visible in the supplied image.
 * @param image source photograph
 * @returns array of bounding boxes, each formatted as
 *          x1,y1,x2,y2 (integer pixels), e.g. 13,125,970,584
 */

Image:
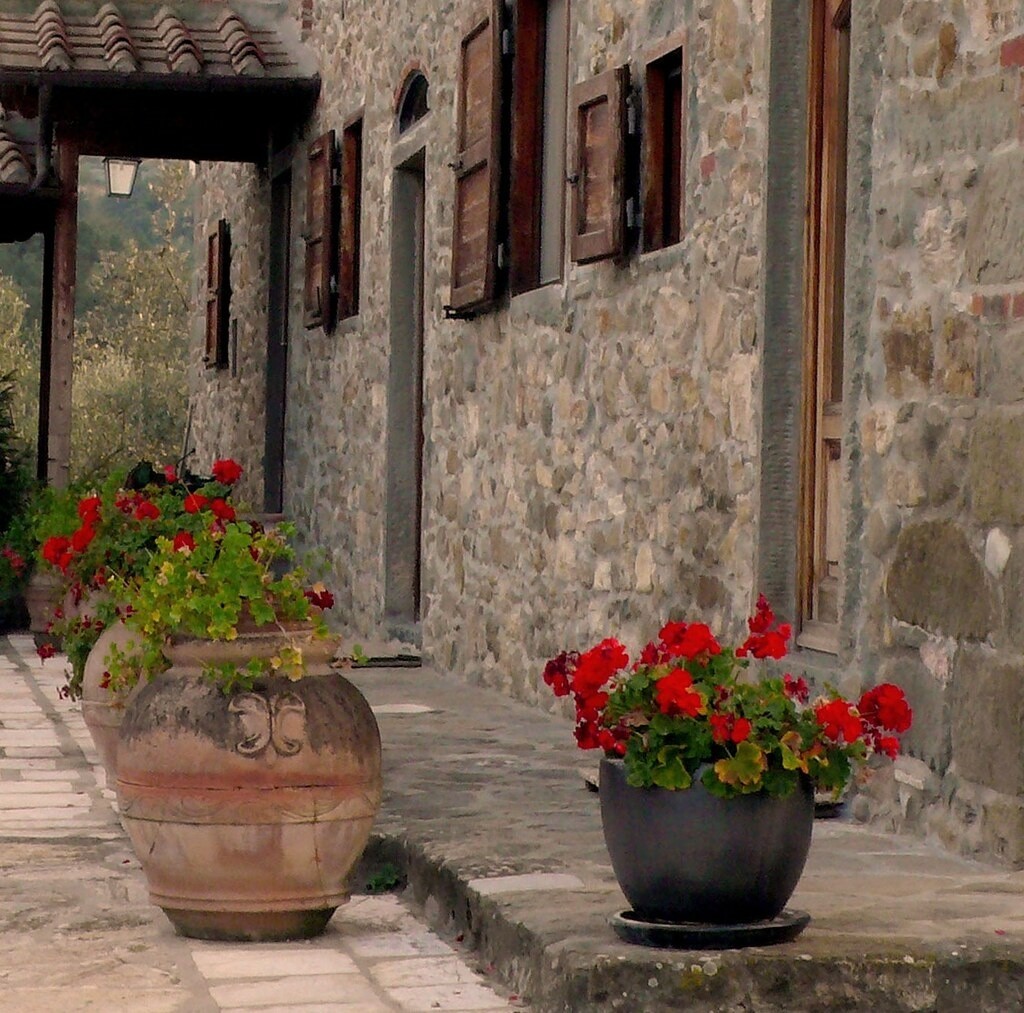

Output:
101,156,143,198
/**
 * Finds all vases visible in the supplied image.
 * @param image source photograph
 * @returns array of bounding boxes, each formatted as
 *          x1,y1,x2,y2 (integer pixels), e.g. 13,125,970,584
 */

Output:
25,565,385,943
596,757,816,952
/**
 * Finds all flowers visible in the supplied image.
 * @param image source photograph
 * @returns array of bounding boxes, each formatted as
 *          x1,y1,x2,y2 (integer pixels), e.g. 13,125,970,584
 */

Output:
0,456,372,707
539,580,911,798
